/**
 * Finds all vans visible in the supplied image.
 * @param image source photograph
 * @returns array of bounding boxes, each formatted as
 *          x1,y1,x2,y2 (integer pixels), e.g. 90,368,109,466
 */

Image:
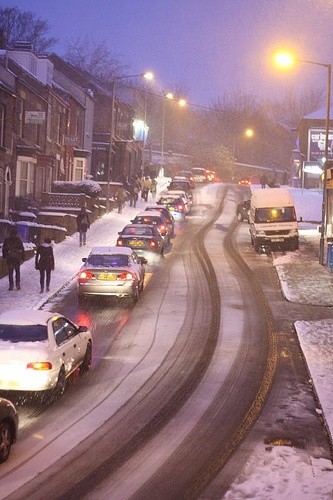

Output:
247,187,303,253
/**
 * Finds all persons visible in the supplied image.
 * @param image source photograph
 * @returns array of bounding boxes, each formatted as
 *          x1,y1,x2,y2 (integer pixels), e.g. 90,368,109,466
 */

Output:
76,207,90,247
141,178,151,202
129,180,139,207
35,238,55,292
150,179,157,199
2,230,24,291
260,174,267,189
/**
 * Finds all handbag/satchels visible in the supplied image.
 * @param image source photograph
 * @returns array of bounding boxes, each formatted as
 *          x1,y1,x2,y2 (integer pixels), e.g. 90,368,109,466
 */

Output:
36,264,40,270
6,250,23,261
134,187,138,194
81,212,89,227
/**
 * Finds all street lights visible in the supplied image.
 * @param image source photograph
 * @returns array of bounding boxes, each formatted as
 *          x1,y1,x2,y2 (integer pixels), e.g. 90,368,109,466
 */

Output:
273,53,333,265
142,92,173,178
161,99,187,178
106,72,154,212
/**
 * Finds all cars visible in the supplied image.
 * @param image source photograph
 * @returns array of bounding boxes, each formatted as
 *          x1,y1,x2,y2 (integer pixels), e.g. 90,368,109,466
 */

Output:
78,246,148,304
0,396,19,464
155,167,216,224
117,224,165,256
130,212,171,248
145,205,175,235
0,310,93,403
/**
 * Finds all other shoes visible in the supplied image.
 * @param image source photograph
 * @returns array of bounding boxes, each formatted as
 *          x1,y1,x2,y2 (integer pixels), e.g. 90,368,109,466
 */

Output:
46,288,50,291
40,290,44,294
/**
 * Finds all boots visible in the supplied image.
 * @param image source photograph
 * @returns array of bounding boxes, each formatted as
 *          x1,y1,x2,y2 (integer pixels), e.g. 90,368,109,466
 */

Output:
8,281,13,290
16,282,22,290
